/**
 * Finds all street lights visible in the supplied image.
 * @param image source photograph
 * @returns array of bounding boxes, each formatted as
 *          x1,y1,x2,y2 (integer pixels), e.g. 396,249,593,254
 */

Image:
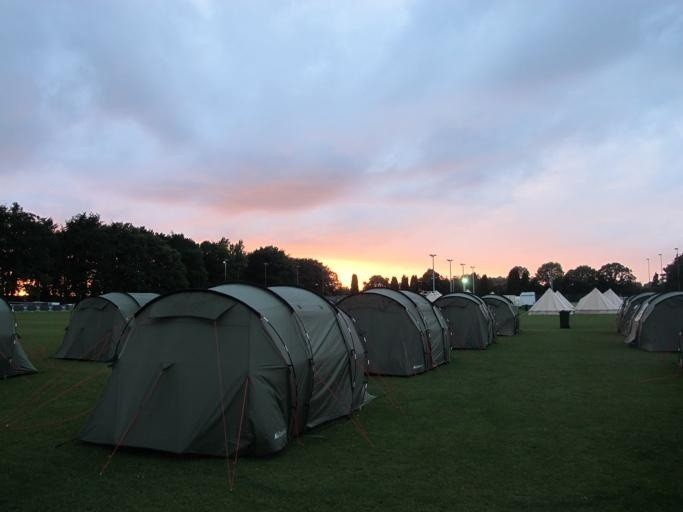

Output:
428,253,437,291
295,264,301,285
674,247,678,257
469,265,476,293
222,259,228,281
645,258,650,287
459,263,466,292
657,253,662,275
446,258,453,292
263,263,268,287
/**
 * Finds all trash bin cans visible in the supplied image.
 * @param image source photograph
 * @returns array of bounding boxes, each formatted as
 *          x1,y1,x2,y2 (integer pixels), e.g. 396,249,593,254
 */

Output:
558,310,571,329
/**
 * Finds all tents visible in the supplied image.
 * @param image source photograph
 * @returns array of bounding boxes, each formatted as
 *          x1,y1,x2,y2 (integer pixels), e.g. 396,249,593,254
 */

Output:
332,288,454,377
626,291,682,352
480,294,518,336
417,289,443,303
526,287,622,317
49,291,161,362
616,291,658,336
0,298,39,380
68,283,379,460
430,292,493,350
